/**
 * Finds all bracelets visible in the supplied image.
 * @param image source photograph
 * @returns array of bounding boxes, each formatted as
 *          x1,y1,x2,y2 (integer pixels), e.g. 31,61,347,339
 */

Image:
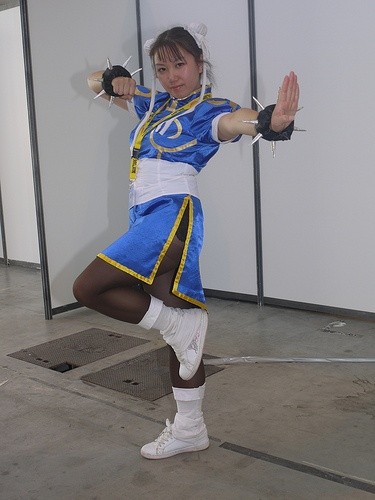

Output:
241,86,307,157
91,55,143,110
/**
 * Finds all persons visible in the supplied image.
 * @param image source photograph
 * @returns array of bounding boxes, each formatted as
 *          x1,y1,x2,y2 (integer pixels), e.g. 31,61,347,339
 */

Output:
71,27,300,460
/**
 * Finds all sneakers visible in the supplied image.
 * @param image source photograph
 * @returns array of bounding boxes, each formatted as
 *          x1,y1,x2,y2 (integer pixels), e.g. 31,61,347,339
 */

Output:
140,418,209,459
168,307,208,380
